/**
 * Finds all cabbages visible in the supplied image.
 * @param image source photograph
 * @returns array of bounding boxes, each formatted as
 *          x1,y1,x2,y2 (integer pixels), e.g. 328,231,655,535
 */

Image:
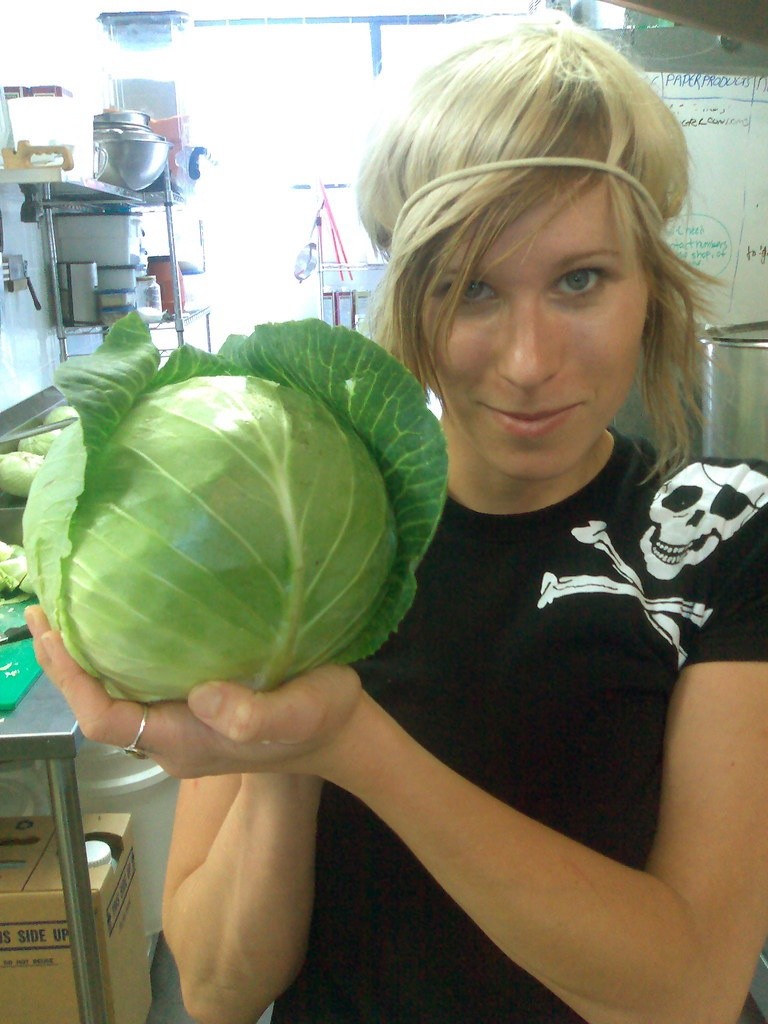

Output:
0,309,450,702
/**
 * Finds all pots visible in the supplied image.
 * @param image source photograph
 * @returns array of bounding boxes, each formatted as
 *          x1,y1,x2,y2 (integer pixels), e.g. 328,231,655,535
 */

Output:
704,324,768,460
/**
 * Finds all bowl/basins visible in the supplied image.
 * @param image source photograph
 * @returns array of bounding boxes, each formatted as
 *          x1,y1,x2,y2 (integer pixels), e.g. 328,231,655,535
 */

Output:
93,110,170,192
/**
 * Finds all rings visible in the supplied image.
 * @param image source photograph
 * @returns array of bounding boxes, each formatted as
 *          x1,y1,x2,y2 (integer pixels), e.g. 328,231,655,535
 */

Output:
125,703,149,759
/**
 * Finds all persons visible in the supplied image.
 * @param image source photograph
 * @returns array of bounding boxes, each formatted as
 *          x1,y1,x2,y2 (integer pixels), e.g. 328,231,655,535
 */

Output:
26,16,767,1024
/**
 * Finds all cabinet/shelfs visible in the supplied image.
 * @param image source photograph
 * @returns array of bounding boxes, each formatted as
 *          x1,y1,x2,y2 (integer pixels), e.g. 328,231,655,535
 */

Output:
0,137,211,364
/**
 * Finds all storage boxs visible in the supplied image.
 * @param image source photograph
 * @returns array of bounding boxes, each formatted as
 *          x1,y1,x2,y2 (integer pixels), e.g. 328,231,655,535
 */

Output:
37,212,144,289
0,813,152,1023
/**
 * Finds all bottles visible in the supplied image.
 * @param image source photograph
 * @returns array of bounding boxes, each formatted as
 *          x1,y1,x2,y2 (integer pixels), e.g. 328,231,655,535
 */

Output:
136,275,163,323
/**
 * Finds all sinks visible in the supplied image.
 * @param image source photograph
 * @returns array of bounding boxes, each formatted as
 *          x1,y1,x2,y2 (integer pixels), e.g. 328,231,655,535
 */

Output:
0,494,28,543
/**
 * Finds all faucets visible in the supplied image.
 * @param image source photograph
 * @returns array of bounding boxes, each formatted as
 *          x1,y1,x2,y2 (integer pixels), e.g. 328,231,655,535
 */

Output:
0,417,79,444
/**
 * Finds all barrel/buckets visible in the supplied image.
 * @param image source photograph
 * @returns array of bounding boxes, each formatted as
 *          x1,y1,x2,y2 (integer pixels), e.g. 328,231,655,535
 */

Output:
75,748,180,937
